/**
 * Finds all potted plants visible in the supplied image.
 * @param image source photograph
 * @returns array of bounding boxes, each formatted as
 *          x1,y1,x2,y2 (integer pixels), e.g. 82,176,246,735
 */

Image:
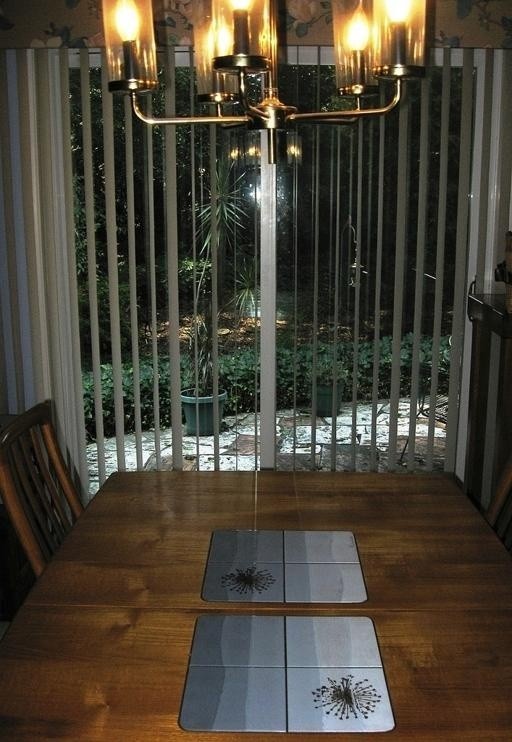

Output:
318,372,347,417
175,150,262,436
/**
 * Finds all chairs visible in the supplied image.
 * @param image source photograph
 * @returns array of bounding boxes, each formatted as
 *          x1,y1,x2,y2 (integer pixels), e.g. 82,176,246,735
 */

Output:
1,401,85,581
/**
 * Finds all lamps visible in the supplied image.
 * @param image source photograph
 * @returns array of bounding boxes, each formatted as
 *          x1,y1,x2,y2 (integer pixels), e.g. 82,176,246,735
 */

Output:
101,0,428,165
